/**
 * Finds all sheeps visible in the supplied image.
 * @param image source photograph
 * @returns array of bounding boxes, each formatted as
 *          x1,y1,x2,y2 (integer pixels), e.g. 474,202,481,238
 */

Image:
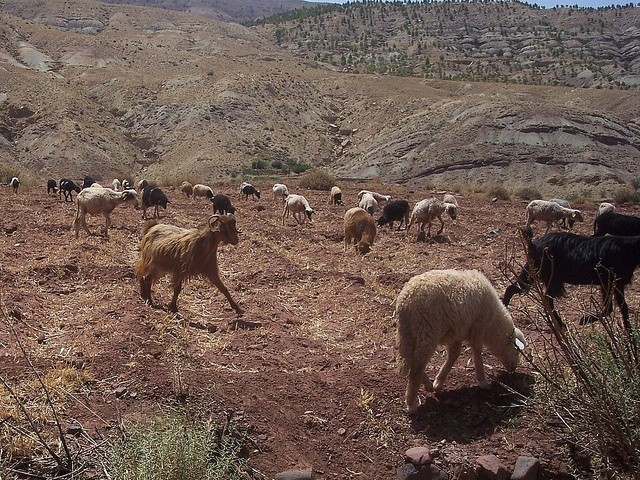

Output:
210,193,237,216
121,178,132,191
597,201,619,217
390,267,529,415
237,181,262,203
59,178,82,203
405,197,459,239
281,194,315,226
82,175,97,189
358,192,380,215
132,210,247,316
70,184,139,240
343,206,379,258
138,179,150,192
357,189,394,207
589,211,640,242
522,198,586,234
9,176,22,195
499,226,640,335
90,182,103,190
192,184,215,202
111,177,122,192
549,196,573,210
180,179,196,198
441,193,463,208
272,183,291,202
139,185,172,220
329,185,344,207
47,179,58,195
376,198,415,233
